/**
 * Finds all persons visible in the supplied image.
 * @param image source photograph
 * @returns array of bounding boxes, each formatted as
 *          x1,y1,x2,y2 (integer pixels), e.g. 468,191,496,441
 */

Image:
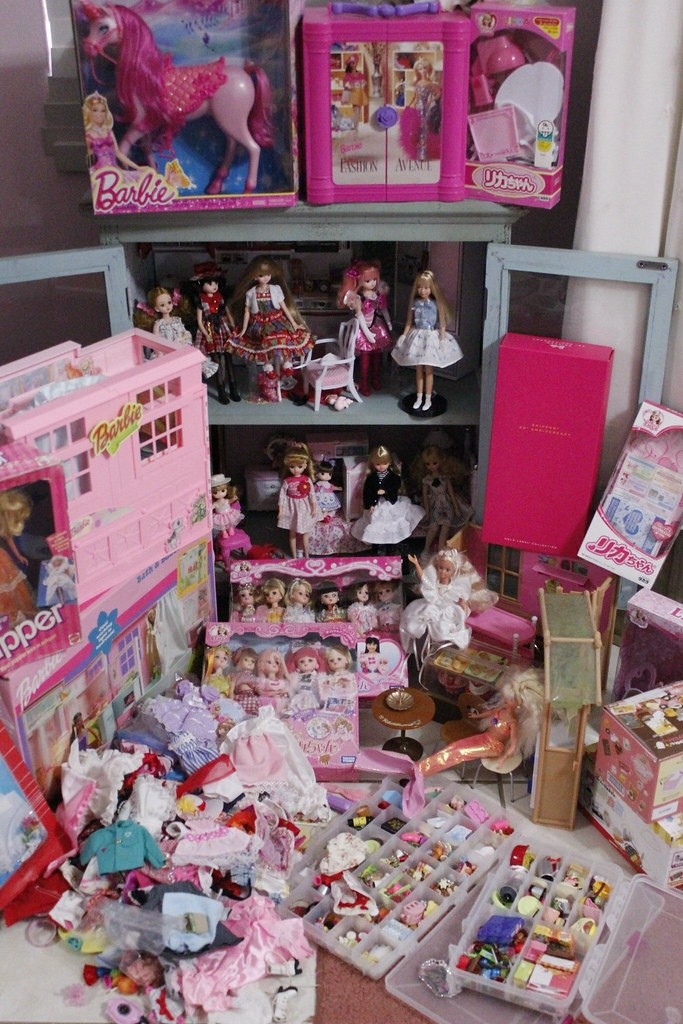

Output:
133,287,220,383
229,647,262,716
350,444,426,556
187,260,241,405
227,255,315,379
209,473,245,539
278,638,353,720
276,441,324,558
231,577,400,635
357,635,404,691
353,665,545,819
204,645,232,700
399,548,499,690
416,447,474,560
255,649,292,719
336,258,394,397
390,270,465,410
0,491,39,628
312,460,343,523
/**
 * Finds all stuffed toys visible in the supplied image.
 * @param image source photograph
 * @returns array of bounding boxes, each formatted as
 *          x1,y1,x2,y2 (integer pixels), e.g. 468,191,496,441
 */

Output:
325,394,353,410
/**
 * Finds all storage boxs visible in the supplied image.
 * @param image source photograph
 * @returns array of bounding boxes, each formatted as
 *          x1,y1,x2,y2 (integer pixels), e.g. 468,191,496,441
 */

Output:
0,440,82,679
301,6,470,206
227,556,407,698
577,400,682,590
0,530,218,792
577,588,682,888
200,621,359,780
463,1,575,208
480,333,615,560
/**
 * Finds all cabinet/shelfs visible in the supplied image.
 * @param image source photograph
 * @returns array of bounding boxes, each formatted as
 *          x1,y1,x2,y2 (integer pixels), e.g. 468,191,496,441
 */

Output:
1,196,679,710
